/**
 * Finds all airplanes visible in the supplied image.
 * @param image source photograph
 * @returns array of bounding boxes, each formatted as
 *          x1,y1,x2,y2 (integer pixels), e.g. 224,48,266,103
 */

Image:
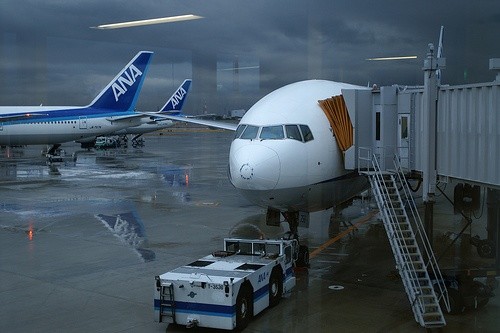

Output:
0,50,191,152
134,80,424,264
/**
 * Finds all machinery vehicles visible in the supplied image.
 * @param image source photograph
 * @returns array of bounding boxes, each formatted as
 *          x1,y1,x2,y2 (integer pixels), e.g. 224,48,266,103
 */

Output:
153,237,299,332
